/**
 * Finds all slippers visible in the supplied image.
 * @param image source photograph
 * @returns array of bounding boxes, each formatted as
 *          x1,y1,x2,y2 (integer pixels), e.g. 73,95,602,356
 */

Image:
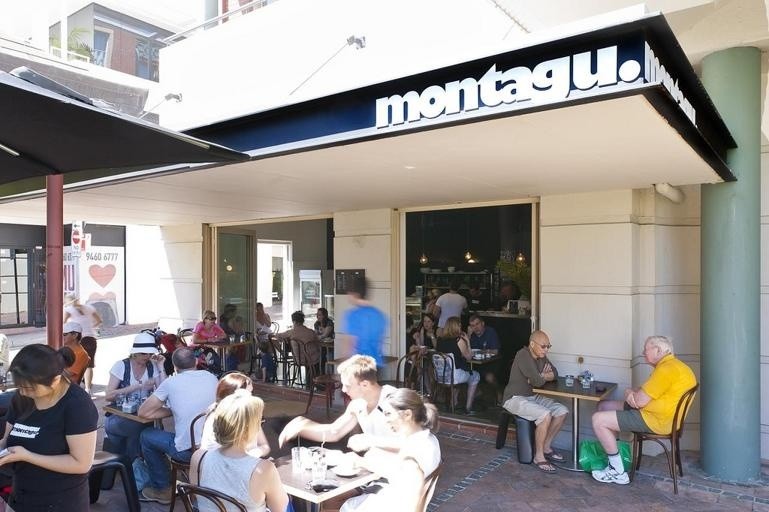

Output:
545,447,567,463
530,461,557,474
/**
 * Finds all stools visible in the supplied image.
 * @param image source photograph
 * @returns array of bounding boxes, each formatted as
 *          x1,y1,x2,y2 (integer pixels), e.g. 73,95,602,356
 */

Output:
89,451,141,512
496,409,536,464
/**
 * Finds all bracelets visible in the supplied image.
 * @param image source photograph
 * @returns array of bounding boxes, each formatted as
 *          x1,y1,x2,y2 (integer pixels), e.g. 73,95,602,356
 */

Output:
159,370,165,373
138,380,142,390
431,336,435,339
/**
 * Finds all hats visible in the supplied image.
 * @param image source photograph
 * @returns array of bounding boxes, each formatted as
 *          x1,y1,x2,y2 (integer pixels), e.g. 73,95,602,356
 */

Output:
64,294,79,306
130,334,158,354
63,322,82,333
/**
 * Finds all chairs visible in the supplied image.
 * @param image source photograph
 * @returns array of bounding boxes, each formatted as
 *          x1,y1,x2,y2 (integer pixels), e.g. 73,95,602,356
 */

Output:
177,483,247,512
630,383,699,494
178,328,195,346
268,333,303,388
249,322,280,373
377,350,419,388
165,412,206,512
304,341,341,418
430,352,465,414
288,338,320,388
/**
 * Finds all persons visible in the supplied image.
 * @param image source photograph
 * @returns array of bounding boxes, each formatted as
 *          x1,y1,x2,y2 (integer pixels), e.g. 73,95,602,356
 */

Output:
279,354,402,472
201,372,274,462
277,311,322,386
190,393,289,512
0,343,99,512
467,315,501,394
0,334,10,392
420,314,437,395
503,330,569,473
190,310,227,378
220,304,242,336
463,283,489,311
62,322,88,385
104,334,168,492
436,316,481,414
314,308,334,368
63,291,103,394
340,388,441,512
499,280,528,313
139,348,219,504
433,278,471,332
256,303,278,357
592,336,697,484
342,277,389,434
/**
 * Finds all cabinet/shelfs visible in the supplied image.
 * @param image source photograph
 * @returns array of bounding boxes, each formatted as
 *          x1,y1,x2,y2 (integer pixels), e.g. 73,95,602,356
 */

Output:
422,272,492,304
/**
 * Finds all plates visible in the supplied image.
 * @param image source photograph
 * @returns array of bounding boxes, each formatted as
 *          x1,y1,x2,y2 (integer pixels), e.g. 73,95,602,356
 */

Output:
332,466,360,476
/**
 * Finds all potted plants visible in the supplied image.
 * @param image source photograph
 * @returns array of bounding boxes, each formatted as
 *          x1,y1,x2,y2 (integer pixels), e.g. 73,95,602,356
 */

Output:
495,248,531,302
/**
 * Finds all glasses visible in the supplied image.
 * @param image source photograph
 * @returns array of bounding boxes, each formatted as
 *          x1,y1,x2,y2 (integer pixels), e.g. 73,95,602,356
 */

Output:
532,340,552,349
206,317,217,321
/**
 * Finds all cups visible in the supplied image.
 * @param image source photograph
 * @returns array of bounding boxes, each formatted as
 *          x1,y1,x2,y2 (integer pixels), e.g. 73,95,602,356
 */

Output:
292,446,308,479
311,449,327,479
115,391,126,407
566,375,574,387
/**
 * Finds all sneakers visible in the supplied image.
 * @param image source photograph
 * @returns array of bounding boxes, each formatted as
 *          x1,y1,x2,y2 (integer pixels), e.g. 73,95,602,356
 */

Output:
142,487,172,504
591,463,630,484
